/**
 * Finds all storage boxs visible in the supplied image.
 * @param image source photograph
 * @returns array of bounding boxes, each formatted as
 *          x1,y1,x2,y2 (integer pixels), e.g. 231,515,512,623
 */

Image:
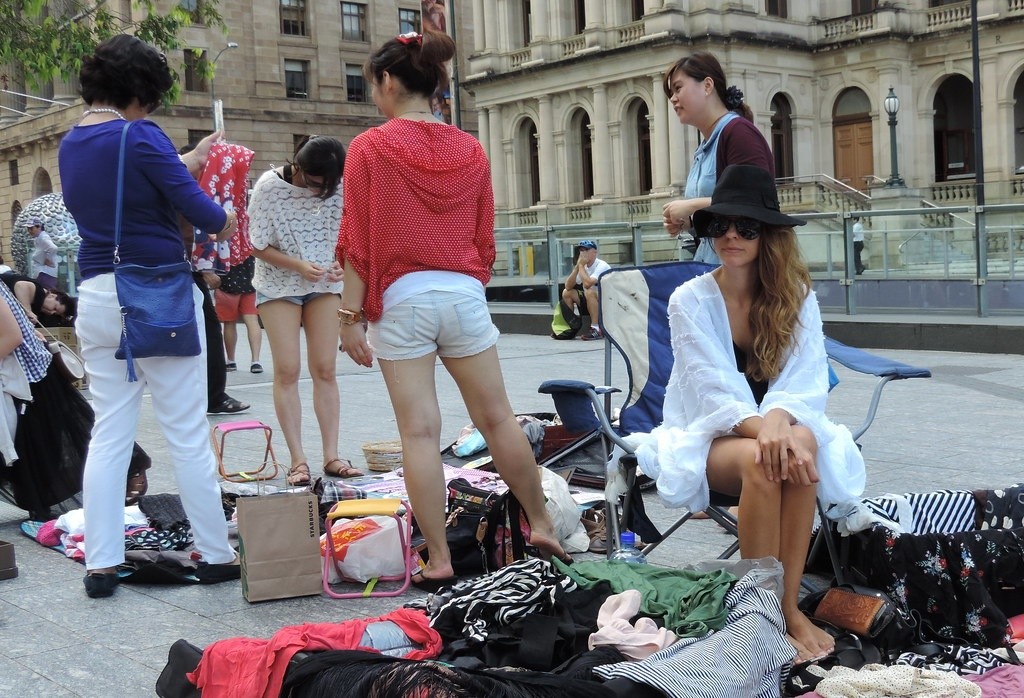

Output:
362,442,405,471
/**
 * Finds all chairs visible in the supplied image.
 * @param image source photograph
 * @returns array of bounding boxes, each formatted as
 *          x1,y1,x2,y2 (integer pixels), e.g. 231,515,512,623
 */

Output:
535,259,930,598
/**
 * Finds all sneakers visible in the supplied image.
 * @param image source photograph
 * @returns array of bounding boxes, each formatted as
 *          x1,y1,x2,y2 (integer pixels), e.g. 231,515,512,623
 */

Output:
581,327,604,340
250,364,263,372
207,397,249,416
225,362,237,372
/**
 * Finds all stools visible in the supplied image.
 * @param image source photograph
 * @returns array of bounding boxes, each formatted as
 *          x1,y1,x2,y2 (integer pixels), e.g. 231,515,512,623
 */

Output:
212,421,278,483
322,497,413,601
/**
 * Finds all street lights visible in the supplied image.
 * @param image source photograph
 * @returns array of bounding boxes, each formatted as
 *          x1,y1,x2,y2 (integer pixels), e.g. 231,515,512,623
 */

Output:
210,42,240,141
882,84,908,189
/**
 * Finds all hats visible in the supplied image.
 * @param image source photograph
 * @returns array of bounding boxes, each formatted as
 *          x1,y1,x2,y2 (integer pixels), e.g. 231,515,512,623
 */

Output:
21,217,45,226
689,161,808,236
575,241,598,250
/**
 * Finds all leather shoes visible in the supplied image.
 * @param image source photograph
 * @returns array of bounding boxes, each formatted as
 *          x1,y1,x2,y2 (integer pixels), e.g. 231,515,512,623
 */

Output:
84,572,120,597
193,561,241,585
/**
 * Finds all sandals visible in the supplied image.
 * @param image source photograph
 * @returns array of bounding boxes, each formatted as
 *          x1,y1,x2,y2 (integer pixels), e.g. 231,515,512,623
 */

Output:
124,469,147,504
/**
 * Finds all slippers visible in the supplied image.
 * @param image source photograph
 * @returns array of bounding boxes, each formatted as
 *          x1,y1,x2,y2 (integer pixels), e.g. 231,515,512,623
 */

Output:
553,550,574,565
410,569,458,593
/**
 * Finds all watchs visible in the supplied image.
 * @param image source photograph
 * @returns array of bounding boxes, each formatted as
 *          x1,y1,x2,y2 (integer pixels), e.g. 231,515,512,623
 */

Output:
337,306,365,326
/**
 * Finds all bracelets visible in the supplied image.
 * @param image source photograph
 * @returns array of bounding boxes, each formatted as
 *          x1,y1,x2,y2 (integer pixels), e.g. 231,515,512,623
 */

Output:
219,213,233,234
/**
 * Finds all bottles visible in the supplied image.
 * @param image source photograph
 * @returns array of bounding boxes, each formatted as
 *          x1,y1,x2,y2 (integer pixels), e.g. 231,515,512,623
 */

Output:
609,532,647,564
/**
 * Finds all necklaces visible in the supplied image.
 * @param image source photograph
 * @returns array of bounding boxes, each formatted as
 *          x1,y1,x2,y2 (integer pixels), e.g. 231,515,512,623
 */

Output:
394,111,433,118
82,108,127,121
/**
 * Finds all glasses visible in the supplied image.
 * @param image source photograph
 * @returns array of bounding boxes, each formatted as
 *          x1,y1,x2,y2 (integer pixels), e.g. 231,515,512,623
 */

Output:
707,213,764,241
299,173,342,189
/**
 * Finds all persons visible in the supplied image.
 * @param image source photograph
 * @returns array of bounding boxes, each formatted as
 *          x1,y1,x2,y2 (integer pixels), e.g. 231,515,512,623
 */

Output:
333,30,578,596
172,144,251,416
629,161,868,669
244,132,364,488
0,270,74,343
57,34,239,600
851,215,866,276
562,239,613,342
661,49,778,270
0,255,13,274
0,292,152,522
199,254,264,375
21,215,59,289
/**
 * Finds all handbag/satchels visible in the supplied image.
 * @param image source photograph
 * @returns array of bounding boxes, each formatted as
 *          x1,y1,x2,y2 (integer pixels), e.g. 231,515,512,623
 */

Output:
447,477,529,571
813,587,896,637
408,511,489,579
235,461,323,604
6,286,85,382
799,581,969,667
111,120,202,383
